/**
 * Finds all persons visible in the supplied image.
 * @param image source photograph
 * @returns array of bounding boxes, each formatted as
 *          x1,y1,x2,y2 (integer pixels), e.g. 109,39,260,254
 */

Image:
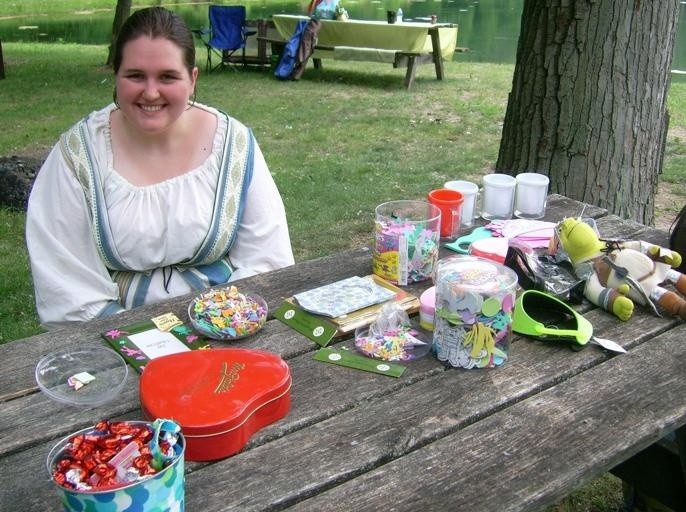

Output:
549,219,686,321
25,7,294,332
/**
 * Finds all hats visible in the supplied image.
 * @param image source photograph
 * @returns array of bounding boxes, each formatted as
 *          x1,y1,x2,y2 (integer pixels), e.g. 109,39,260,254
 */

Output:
509,282,594,349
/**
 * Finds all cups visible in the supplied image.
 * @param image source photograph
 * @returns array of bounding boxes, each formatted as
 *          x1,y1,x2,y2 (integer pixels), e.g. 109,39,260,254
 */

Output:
387,10,396,24
427,188,464,237
443,180,479,230
481,173,516,222
518,172,550,218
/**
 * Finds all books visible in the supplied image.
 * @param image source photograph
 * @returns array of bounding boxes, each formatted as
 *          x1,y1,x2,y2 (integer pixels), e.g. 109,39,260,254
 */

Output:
285,274,421,337
98,311,212,377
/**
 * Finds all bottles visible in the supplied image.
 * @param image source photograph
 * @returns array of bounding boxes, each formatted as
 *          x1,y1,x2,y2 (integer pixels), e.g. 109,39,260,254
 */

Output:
429,254,519,373
371,197,443,288
396,7,403,24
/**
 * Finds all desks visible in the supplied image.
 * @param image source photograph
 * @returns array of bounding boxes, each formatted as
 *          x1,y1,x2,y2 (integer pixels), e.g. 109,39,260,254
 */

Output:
0,194,686,509
269,14,459,91
221,18,273,68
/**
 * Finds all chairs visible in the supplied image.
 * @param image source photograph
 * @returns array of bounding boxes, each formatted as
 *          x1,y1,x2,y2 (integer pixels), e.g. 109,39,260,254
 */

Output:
192,5,257,75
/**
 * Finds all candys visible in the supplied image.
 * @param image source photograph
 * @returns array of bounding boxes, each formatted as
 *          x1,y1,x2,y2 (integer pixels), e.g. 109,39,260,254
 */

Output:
54,420,177,490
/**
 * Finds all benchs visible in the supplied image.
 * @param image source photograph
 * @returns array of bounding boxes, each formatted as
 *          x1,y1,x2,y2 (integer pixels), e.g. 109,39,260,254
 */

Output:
258,36,471,89
